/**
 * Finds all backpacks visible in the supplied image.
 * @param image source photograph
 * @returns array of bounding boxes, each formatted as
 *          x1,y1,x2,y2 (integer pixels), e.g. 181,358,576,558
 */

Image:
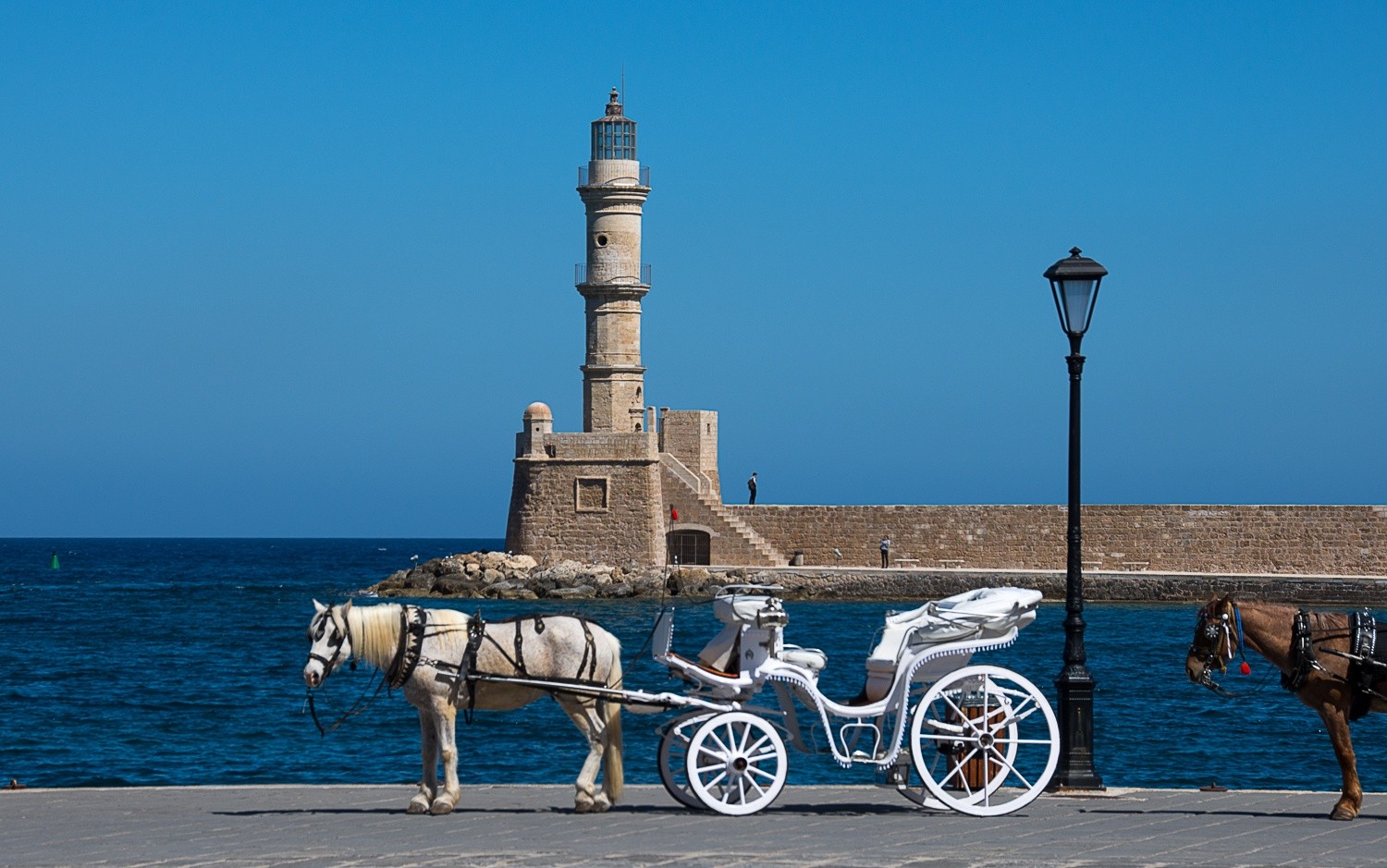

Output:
748,478,755,490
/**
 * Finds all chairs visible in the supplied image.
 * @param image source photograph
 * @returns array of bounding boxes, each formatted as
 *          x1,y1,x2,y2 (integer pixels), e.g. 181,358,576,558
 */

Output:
866,587,1043,697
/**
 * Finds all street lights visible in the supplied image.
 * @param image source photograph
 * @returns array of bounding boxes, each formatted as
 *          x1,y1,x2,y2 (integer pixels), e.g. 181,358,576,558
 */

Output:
1042,246,1108,793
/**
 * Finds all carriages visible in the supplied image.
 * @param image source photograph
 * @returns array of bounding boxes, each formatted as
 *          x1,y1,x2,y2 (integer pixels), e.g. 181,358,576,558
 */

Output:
304,585,1060,816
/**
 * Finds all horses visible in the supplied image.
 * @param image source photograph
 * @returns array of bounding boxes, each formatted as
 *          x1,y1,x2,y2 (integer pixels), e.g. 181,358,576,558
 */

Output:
1185,594,1387,822
304,598,625,817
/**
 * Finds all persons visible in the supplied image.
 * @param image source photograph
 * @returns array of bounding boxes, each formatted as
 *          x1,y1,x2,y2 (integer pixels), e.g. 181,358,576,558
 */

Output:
747,472,758,506
879,535,889,568
669,502,678,524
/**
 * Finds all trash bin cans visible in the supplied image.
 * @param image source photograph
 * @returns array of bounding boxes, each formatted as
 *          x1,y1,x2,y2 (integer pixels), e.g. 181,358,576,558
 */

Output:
946,696,1004,791
794,552,804,565
